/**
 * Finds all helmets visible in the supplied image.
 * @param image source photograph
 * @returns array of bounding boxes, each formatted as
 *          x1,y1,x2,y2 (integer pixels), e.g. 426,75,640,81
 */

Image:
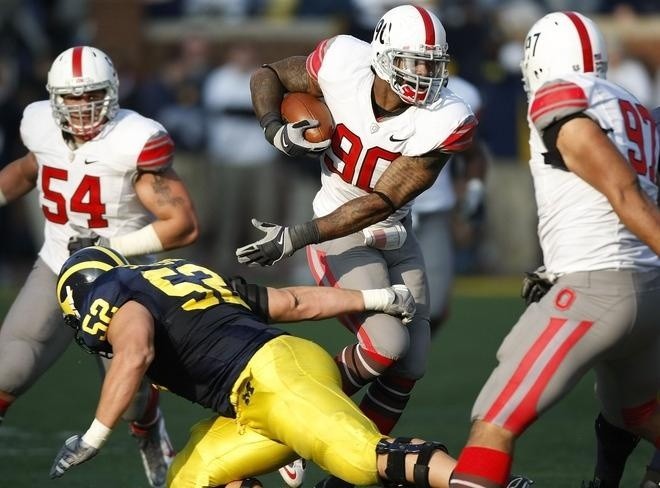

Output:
46,46,120,141
56,246,131,360
520,11,609,101
370,4,450,108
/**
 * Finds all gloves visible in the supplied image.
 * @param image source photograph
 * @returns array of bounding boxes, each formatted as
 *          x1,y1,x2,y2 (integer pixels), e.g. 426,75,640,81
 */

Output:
49,435,102,480
236,219,295,269
522,265,557,303
262,118,331,160
67,222,111,255
384,284,416,325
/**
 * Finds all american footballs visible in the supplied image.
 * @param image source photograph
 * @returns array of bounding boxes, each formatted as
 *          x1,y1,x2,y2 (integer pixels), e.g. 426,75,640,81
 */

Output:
279,92,334,143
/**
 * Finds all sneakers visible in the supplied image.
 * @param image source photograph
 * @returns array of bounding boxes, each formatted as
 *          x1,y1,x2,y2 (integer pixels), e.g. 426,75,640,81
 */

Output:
128,407,177,488
275,458,307,488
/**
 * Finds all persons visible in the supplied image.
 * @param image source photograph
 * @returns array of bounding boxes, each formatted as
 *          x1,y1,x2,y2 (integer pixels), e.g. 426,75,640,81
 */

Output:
2,1,660,345
234,4,481,488
583,316,659,487
48,245,535,487
0,46,195,444
447,9,660,487
